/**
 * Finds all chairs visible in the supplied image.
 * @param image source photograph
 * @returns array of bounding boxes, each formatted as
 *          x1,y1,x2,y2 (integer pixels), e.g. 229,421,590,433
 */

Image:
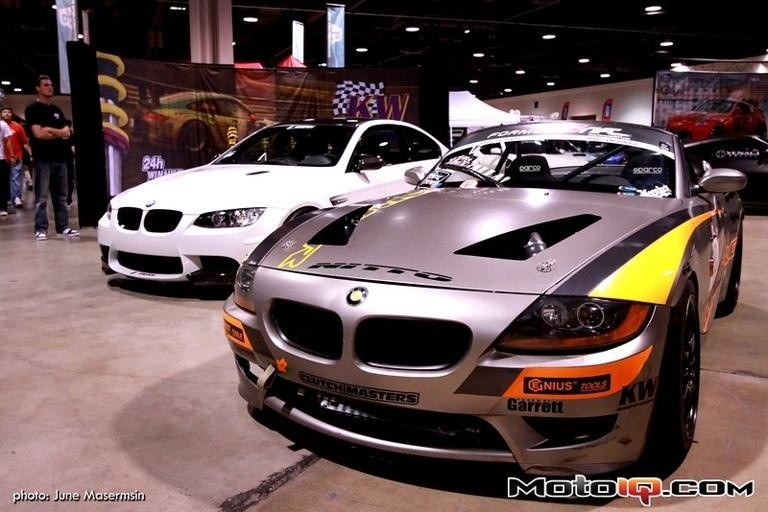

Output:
619,155,668,192
505,155,552,185
301,132,423,164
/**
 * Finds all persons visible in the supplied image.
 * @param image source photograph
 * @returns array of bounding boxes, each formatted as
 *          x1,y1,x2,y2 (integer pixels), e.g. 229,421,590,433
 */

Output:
0,107,32,216
23,74,80,240
63,117,75,210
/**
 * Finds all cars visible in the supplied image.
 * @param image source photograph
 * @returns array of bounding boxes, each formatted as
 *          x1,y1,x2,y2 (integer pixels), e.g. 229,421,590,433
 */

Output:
667,96,766,141
91,114,451,293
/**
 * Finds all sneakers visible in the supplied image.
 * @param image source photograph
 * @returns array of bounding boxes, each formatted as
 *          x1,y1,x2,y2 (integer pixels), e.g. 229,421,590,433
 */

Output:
13,197,23,207
57,227,80,236
34,232,47,240
0,210,9,216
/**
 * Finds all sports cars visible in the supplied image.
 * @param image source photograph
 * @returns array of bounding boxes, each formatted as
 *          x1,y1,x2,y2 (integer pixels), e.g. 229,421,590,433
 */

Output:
135,89,278,153
222,122,748,478
